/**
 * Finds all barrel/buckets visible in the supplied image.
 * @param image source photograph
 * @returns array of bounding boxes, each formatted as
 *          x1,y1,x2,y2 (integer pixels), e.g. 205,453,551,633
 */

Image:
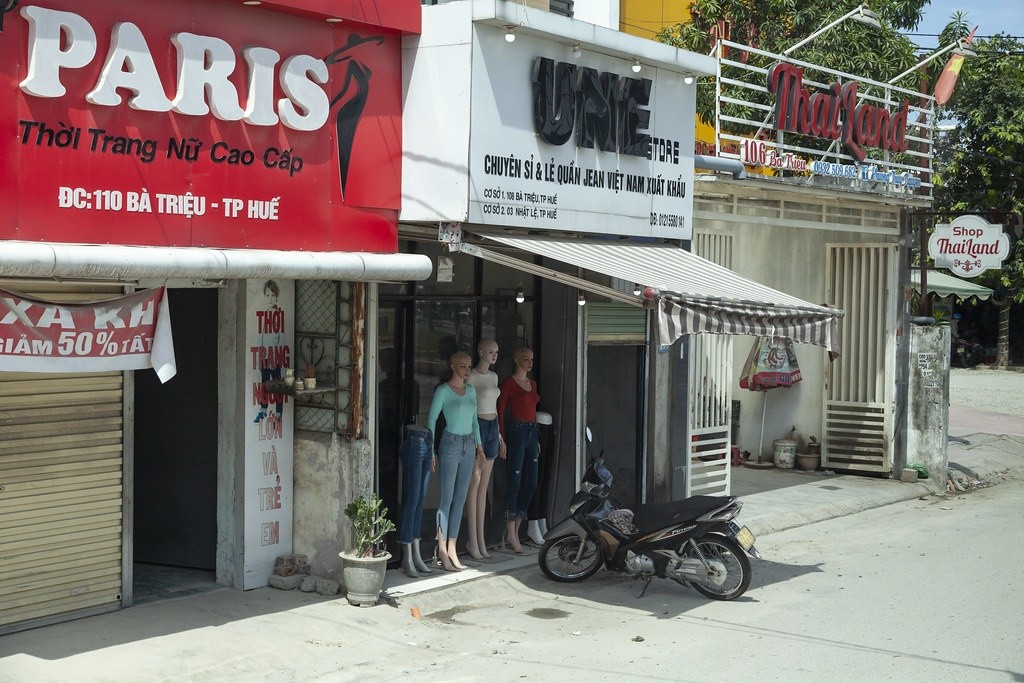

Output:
773,439,798,468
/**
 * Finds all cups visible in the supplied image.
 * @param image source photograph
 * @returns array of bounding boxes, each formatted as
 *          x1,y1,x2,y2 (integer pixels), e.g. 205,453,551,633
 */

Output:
303,377,316,389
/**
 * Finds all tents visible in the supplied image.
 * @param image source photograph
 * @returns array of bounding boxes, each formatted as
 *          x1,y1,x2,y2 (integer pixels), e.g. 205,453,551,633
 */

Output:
911,269,995,300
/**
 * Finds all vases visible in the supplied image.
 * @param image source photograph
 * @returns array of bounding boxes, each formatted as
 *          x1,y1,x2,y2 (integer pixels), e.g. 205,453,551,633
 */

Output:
303,377,317,389
284,368,295,386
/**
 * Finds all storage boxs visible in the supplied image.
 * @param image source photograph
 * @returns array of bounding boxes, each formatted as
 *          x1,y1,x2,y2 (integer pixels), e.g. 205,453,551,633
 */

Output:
699,398,740,422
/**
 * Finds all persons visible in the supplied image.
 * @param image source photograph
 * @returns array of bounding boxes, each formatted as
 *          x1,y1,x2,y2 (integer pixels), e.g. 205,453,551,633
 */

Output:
396,425,432,578
528,412,554,545
465,339,504,560
427,351,486,571
498,347,540,553
253,280,285,429
949,313,962,355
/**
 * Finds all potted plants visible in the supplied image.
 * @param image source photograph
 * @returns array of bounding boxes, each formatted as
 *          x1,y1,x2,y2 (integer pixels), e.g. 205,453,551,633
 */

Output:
796,441,821,470
809,435,821,454
336,492,397,608
772,424,799,469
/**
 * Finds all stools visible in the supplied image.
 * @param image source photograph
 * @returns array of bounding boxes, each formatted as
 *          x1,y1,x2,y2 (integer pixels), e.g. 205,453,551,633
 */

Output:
718,444,741,467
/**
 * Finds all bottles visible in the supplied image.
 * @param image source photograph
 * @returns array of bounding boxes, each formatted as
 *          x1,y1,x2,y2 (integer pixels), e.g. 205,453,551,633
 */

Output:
284,368,295,386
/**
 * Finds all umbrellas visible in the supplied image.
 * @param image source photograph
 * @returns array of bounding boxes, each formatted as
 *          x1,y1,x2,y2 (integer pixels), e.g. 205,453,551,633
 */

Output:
739,334,802,457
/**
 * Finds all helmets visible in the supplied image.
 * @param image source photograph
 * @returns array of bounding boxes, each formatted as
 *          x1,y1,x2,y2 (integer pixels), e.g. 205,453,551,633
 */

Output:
952,313,961,319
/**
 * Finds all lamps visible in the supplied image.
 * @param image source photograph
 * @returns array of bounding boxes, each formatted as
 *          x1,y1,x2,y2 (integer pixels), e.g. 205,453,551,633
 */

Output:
515,282,525,304
632,57,642,73
572,44,581,58
578,289,586,306
504,26,518,43
633,283,641,296
684,71,693,85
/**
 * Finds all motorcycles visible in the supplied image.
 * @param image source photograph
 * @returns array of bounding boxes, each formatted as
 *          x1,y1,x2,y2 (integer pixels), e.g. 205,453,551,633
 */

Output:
538,428,761,600
955,322,982,368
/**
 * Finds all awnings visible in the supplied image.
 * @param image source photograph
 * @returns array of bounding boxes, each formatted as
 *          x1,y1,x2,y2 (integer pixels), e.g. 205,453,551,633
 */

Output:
458,231,845,351
